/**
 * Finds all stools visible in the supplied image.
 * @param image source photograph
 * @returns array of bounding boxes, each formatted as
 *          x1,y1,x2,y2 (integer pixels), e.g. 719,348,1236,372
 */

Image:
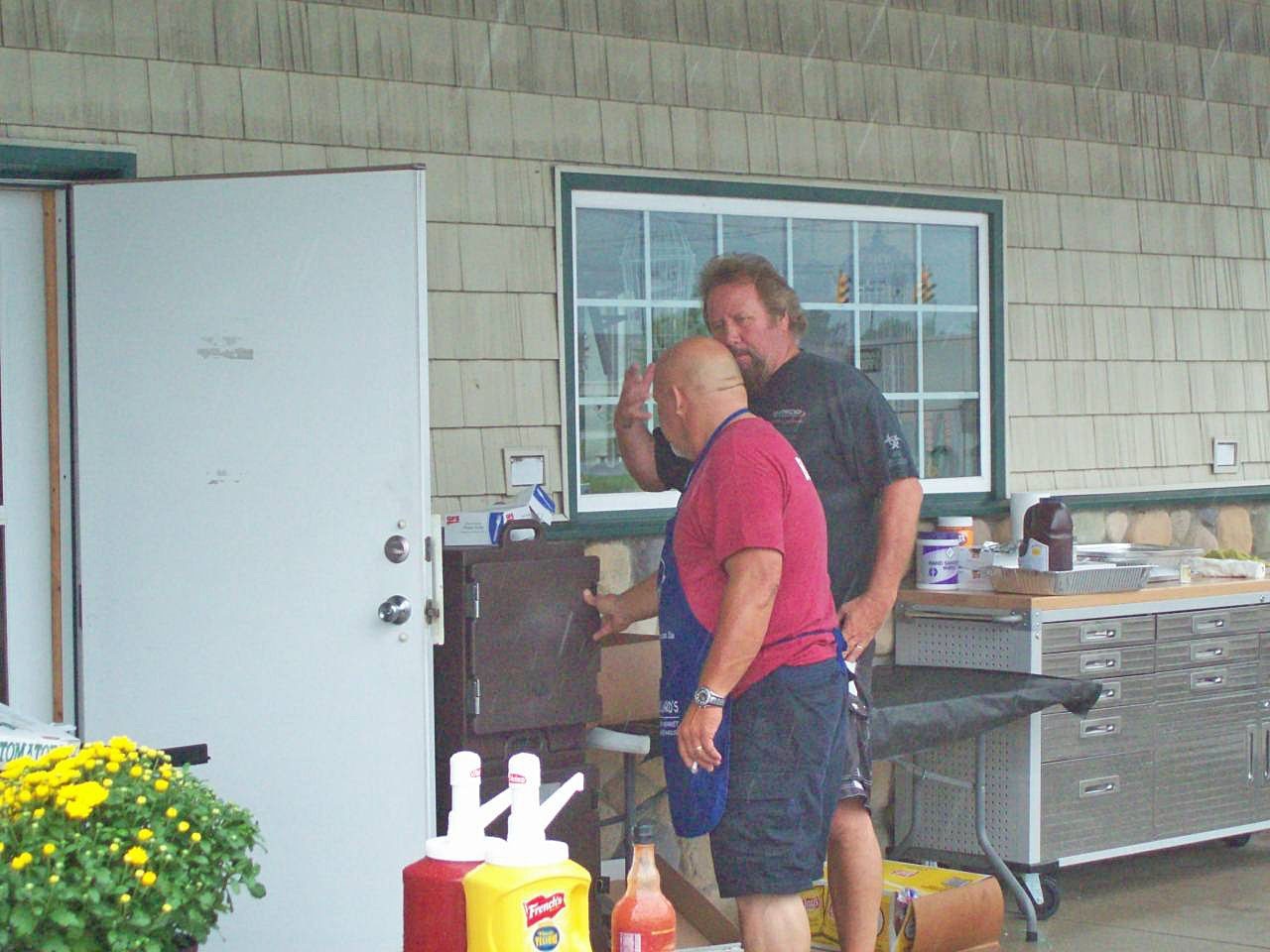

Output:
588,719,667,894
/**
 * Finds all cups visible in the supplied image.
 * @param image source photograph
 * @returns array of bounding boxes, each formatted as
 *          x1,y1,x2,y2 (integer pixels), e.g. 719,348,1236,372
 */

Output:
1010,491,1040,549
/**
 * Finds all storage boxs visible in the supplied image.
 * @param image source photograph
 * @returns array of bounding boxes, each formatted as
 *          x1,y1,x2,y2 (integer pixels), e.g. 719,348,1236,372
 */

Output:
1075,544,1204,580
599,633,661,726
606,853,743,952
799,857,1005,952
442,483,557,546
990,562,1158,597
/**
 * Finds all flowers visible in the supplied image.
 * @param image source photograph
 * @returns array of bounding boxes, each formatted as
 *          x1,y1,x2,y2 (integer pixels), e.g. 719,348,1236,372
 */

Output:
0,735,266,952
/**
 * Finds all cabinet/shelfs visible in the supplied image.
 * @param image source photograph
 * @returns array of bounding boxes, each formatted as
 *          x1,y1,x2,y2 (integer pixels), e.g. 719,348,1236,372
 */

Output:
434,522,600,952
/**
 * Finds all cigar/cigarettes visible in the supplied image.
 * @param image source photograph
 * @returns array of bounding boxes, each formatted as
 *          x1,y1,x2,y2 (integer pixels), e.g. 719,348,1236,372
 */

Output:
690,762,698,773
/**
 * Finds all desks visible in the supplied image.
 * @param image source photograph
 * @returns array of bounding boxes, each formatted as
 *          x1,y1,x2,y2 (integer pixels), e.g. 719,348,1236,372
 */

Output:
587,664,1102,942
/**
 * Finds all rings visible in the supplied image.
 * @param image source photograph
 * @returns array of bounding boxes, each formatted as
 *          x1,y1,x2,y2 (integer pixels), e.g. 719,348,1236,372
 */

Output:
856,644,863,650
692,745,702,751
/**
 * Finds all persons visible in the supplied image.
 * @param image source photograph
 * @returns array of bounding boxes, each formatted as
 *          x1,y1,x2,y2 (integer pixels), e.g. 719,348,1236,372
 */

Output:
583,334,848,952
613,250,924,952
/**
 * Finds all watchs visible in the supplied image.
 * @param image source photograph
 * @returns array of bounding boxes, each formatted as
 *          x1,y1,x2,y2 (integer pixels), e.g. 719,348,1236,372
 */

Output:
694,686,726,707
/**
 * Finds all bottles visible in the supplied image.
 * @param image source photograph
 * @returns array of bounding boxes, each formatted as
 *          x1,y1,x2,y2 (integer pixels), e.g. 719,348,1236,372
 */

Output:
914,531,960,591
610,825,677,952
934,516,974,547
462,752,593,952
401,748,511,952
